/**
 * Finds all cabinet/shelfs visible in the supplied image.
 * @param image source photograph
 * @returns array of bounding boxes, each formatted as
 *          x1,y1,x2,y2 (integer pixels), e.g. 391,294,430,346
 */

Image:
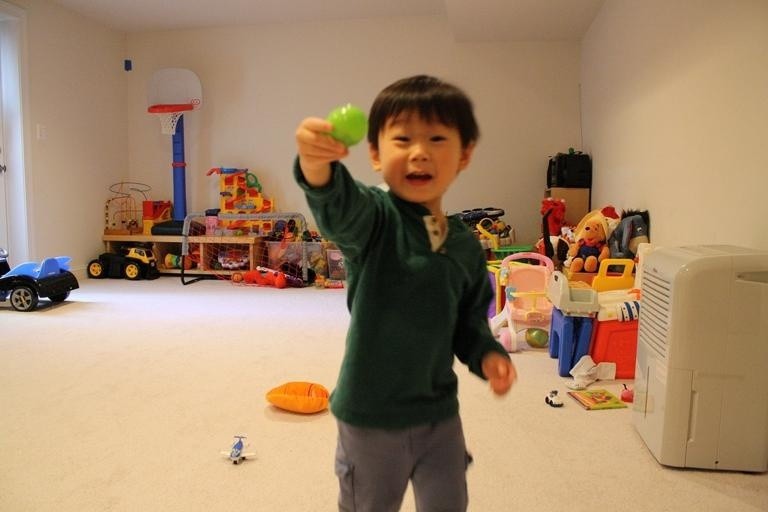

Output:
102,234,265,279
544,188,589,228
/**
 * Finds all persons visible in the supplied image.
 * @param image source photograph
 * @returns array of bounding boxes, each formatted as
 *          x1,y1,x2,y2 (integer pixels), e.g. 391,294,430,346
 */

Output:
293,73,517,511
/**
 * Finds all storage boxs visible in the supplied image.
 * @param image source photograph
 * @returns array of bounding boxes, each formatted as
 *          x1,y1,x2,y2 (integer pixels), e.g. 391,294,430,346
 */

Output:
266,241,347,281
